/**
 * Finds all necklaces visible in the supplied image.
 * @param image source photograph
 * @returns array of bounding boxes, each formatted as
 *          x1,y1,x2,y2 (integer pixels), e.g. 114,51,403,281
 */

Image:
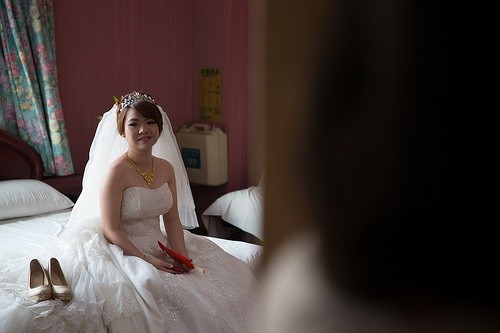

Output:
126,152,157,186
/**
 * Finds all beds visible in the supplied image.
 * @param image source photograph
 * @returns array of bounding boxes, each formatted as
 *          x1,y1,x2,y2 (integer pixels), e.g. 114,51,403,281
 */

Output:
0,129,262,333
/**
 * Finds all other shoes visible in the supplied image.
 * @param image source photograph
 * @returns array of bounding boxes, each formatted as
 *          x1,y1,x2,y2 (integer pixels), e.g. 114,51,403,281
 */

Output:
28,259,52,301
47,257,74,302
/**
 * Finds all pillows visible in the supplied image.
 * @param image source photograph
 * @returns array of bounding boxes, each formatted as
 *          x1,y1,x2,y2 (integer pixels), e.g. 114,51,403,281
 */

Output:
0,178,74,220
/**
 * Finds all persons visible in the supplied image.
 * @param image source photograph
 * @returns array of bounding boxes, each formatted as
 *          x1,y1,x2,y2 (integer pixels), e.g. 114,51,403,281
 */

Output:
70,92,245,333
